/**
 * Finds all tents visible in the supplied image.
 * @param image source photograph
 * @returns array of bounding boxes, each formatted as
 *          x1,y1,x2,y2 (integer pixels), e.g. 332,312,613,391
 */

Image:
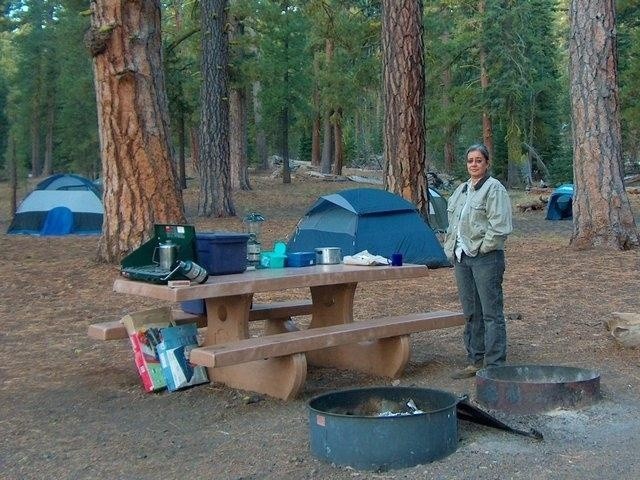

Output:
284,187,456,268
544,183,573,221
425,185,450,231
4,172,105,237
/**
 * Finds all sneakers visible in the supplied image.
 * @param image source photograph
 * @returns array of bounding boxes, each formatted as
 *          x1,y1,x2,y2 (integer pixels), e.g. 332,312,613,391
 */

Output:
452,366,478,379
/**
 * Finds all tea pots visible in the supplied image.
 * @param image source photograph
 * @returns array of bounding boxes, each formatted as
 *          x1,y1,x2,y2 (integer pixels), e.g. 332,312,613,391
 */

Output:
152,240,181,270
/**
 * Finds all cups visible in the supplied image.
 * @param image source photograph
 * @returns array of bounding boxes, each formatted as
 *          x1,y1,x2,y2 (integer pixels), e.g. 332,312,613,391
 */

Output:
386,253,402,266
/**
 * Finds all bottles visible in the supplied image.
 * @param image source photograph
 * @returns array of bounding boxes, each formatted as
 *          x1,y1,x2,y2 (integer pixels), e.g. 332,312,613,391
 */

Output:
176,259,209,284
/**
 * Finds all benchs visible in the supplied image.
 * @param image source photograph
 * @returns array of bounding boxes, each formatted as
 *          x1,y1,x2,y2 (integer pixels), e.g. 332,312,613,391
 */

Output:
188,310,467,366
86,301,314,343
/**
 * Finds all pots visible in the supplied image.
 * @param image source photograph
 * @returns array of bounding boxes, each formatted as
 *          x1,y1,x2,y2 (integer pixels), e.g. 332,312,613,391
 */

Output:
313,247,342,265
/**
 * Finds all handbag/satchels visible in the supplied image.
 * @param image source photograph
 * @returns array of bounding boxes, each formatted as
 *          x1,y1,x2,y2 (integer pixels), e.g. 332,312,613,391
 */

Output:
156,339,208,392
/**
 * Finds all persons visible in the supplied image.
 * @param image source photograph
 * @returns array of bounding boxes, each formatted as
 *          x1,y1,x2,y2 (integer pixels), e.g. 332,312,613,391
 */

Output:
442,141,514,383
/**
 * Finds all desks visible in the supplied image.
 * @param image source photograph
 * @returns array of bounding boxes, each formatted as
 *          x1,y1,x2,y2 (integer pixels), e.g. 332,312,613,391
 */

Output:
114,259,428,402
